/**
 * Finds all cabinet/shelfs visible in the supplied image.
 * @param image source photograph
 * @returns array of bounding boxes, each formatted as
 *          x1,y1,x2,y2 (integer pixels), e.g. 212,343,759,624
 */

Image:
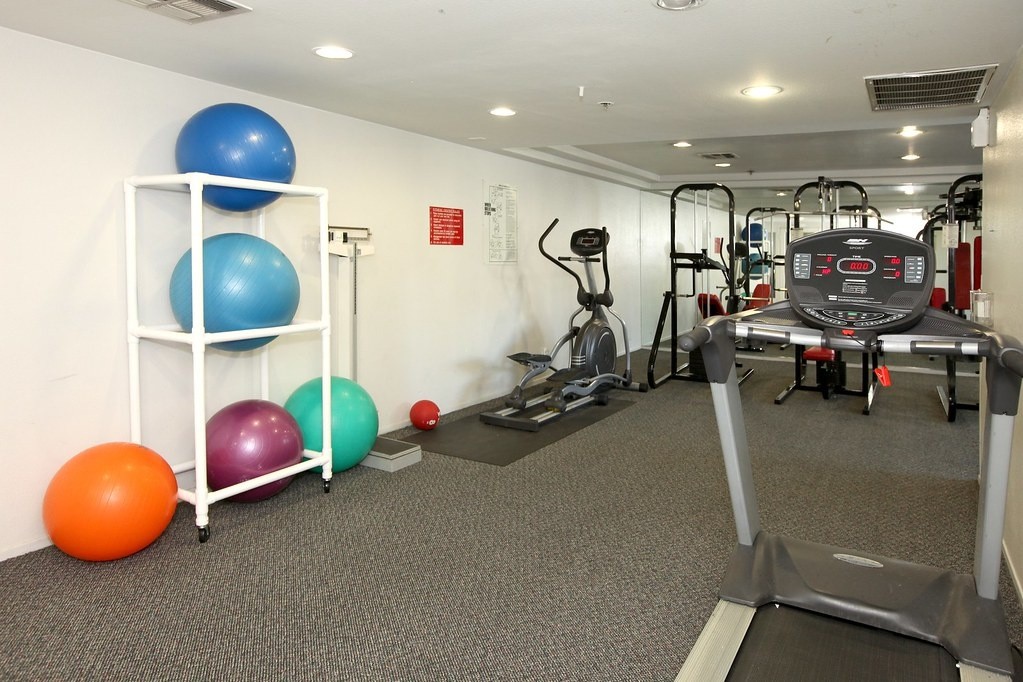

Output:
124,171,334,543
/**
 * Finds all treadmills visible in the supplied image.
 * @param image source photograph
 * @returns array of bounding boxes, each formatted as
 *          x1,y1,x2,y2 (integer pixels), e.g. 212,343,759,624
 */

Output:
667,228,1023,682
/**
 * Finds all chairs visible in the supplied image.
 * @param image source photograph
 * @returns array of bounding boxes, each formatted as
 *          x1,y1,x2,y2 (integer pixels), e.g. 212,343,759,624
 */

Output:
742,283,773,314
696,294,731,323
929,287,947,311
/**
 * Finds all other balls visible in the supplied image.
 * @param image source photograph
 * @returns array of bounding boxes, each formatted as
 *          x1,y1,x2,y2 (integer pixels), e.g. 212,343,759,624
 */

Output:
205,399,303,504
741,222,766,247
283,375,379,473
170,233,300,352
411,401,439,430
176,101,296,213
43,439,179,563
741,253,768,280
